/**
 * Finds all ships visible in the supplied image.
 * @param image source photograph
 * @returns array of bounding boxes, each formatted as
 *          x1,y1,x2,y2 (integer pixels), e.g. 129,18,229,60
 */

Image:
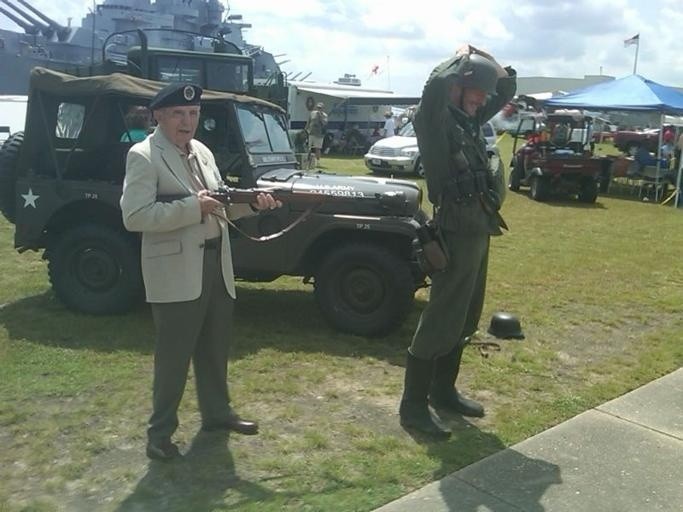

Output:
0,0,393,106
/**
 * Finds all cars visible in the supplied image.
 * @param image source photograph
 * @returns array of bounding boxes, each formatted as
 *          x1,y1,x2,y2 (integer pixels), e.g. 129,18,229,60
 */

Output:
614,124,683,156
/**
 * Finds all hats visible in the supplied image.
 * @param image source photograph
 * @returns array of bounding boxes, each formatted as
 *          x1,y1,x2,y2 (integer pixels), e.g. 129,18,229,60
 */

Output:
384,111,391,117
316,102,324,108
149,81,202,109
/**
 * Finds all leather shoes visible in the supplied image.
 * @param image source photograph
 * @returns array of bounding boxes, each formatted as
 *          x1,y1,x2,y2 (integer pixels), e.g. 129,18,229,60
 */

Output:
147,440,186,465
201,408,259,434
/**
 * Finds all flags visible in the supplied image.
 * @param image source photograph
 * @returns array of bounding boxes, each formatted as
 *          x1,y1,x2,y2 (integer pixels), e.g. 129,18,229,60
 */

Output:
623,34,638,47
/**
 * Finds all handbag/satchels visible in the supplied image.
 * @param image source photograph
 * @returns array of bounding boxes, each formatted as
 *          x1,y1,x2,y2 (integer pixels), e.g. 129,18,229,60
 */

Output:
416,219,449,271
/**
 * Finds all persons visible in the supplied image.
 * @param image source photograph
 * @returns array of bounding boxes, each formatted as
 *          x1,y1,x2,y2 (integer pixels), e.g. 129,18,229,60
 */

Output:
397,42,518,435
117,106,150,144
117,80,285,462
381,109,396,138
302,100,328,168
635,138,668,170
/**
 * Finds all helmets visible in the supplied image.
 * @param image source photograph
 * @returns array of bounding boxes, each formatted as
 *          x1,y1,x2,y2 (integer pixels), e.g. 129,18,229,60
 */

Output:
452,53,499,96
488,311,524,338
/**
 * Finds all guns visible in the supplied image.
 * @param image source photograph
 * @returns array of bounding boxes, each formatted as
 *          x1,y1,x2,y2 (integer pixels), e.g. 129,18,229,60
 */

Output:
153,178,388,213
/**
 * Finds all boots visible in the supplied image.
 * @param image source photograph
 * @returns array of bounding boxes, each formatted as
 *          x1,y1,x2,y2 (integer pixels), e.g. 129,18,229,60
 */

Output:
430,348,484,417
399,349,453,438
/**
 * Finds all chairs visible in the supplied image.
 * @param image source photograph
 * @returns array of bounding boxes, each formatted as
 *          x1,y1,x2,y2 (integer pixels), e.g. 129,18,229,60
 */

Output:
608,156,665,200
